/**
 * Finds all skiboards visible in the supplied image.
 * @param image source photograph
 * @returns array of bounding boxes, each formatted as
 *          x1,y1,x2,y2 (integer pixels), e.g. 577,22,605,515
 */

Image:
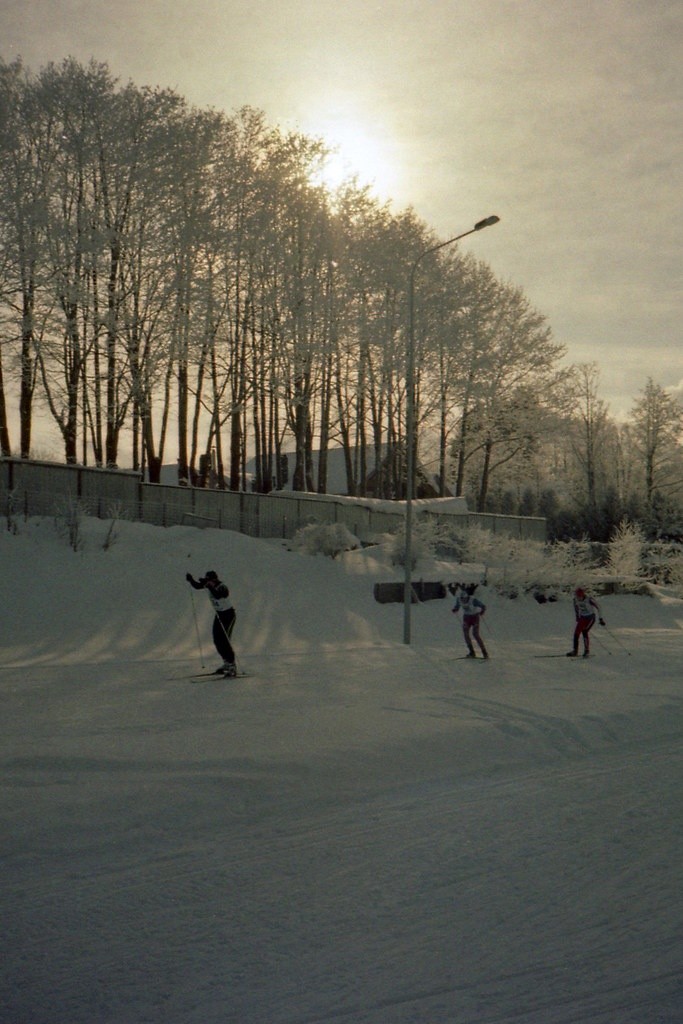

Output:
164,670,256,682
445,656,489,664
535,654,596,661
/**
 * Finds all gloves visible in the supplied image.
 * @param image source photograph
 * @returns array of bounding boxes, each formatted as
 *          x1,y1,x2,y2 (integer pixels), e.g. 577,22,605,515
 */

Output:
598,617,606,626
480,611,485,615
199,578,209,586
452,608,459,612
186,573,193,581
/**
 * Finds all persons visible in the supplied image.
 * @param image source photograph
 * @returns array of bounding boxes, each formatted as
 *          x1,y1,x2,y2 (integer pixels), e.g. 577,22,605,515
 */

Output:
185,571,237,679
451,591,491,660
566,588,606,659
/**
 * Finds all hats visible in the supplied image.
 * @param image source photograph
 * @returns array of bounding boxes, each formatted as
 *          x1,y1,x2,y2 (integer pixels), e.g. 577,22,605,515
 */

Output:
455,590,468,598
206,571,218,581
575,588,584,597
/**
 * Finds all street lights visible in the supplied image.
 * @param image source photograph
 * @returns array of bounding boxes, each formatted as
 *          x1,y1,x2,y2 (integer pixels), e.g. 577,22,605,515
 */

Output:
402,215,503,644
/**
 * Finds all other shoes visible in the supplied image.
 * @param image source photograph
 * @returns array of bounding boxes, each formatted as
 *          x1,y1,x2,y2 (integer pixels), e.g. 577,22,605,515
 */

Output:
567,650,578,656
216,662,233,674
481,649,489,659
224,663,236,676
467,650,475,658
583,650,590,657
